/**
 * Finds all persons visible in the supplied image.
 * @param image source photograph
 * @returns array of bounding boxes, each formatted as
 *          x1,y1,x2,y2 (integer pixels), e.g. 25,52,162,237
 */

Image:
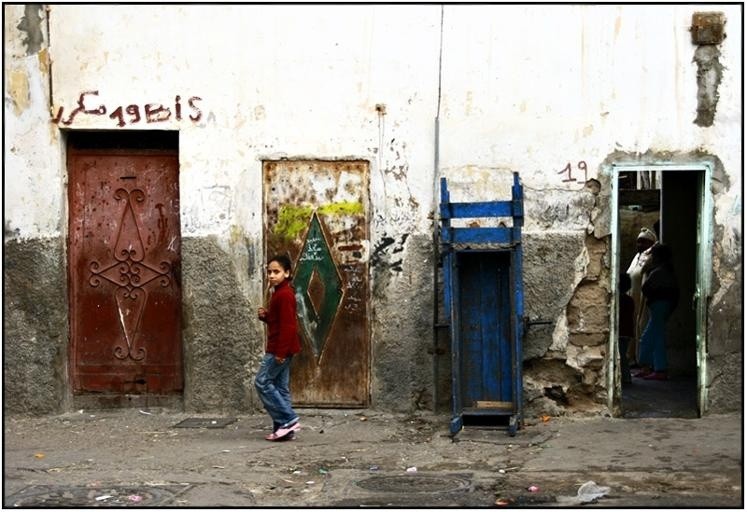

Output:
620,227,679,384
255,256,301,441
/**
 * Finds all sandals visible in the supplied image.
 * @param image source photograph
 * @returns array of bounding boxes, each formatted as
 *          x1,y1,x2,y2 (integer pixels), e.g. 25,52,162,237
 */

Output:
264,421,301,441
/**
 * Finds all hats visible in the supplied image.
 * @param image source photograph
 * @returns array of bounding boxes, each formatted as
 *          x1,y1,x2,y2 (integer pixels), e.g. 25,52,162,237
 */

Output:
637,228,656,242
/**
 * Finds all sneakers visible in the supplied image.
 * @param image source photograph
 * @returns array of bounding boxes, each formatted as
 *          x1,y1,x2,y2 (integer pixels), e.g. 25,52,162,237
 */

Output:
631,369,672,381
